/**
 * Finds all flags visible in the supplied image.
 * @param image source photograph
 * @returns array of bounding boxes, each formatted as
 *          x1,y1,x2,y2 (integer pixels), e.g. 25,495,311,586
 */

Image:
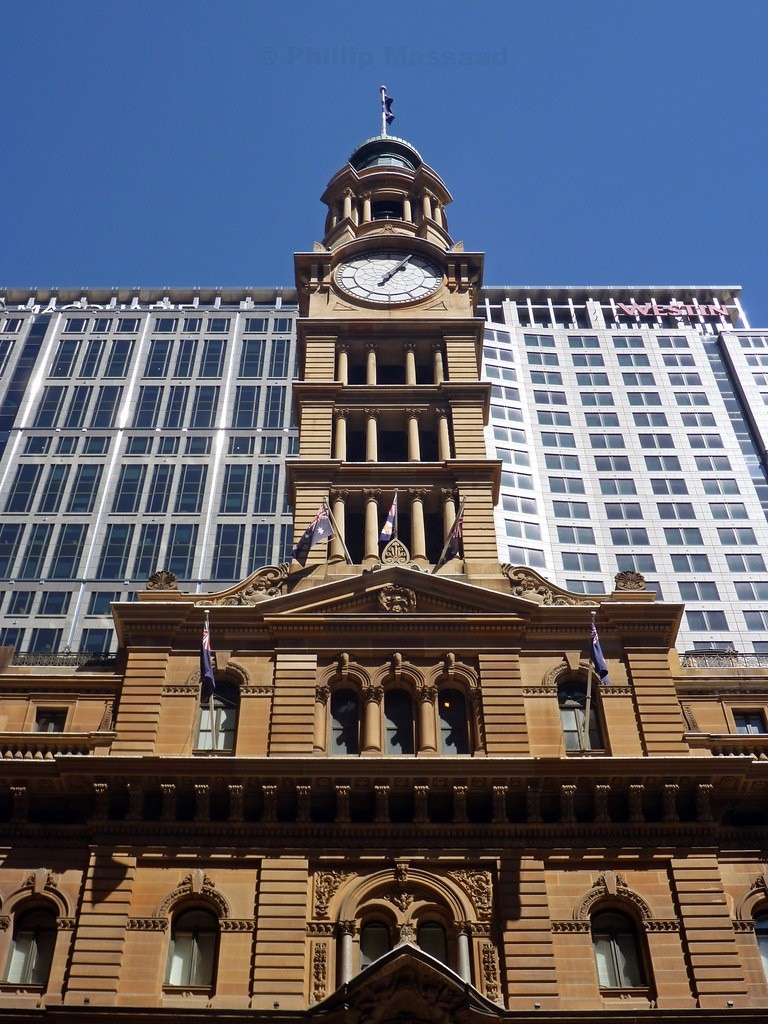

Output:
445,502,465,561
590,623,609,679
200,618,216,702
290,503,335,568
377,492,398,546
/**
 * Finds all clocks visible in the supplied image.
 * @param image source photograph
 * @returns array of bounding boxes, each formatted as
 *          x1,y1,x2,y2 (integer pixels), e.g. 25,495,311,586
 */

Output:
335,250,444,305
